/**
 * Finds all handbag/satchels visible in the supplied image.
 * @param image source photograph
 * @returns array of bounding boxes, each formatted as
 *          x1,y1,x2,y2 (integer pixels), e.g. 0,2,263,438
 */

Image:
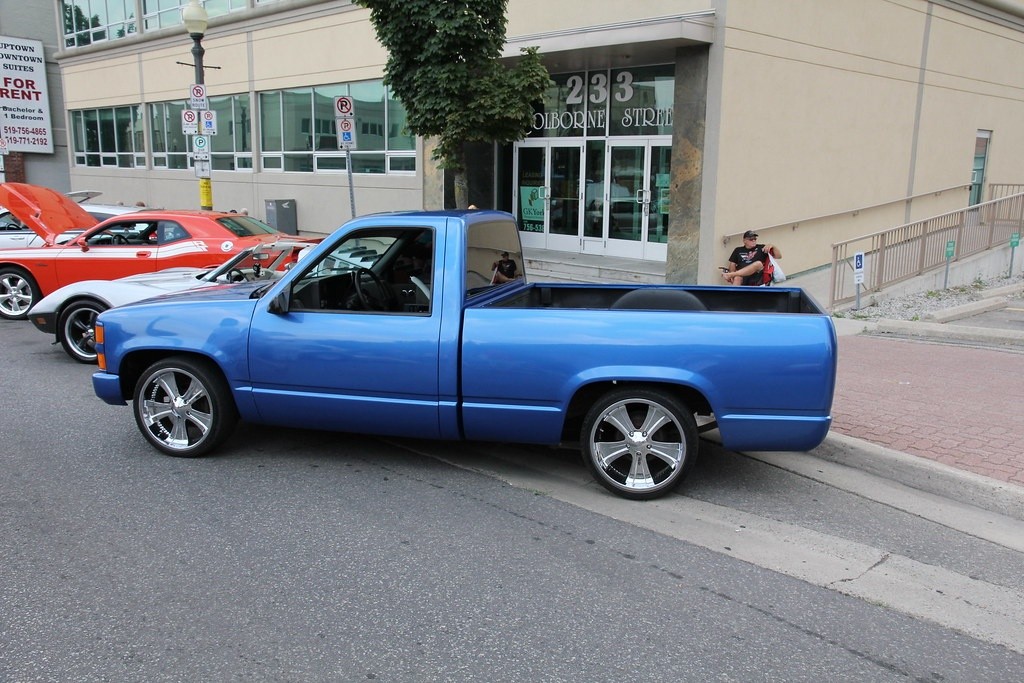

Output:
768,253,786,283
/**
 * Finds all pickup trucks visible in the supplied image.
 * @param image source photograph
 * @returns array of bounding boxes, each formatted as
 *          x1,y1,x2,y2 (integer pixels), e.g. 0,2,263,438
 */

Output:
94,210,836,500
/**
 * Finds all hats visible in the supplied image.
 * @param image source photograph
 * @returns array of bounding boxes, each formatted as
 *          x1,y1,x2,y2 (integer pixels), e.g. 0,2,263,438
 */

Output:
501,252,509,256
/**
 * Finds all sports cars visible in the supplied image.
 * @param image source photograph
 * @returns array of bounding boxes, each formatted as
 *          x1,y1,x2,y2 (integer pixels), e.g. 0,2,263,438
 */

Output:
27,242,384,363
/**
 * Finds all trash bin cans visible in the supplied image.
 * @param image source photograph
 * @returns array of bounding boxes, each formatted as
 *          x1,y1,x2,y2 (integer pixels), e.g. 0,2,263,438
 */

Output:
265,199,297,235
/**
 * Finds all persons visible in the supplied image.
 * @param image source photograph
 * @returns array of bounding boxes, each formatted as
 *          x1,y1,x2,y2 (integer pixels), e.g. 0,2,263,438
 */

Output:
722,230,783,286
491,251,517,283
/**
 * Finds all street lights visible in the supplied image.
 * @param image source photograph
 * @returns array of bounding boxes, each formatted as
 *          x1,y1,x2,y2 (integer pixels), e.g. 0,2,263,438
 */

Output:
178,0,220,210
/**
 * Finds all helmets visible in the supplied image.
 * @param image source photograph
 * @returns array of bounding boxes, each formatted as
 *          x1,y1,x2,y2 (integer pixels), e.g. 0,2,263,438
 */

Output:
743,230,758,238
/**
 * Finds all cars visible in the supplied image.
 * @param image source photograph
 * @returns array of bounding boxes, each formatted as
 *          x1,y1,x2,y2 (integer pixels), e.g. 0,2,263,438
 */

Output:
0,182,325,319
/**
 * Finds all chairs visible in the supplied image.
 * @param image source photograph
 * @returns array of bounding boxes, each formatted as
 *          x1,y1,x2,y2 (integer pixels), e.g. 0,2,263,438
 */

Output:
719,251,775,286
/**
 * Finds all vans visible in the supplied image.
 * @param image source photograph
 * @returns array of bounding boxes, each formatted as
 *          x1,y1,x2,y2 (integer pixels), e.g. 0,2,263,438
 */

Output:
587,198,658,228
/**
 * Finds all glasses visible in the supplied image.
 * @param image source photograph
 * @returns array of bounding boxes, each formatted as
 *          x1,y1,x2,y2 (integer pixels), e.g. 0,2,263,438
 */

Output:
747,238,757,241
